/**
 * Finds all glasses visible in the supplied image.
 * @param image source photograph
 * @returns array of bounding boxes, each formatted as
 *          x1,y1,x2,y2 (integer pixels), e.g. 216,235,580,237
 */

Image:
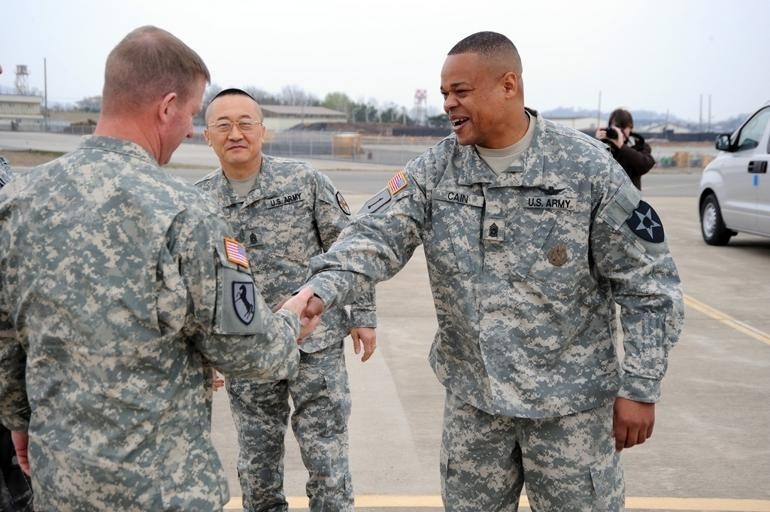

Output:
208,118,261,137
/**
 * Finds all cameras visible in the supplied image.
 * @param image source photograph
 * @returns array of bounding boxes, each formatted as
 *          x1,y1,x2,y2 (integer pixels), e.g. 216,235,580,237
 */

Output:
601,128,618,139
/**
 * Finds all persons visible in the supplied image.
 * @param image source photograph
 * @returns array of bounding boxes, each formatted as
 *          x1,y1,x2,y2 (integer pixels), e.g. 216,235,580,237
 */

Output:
190,87,379,512
2,23,323,512
591,107,657,194
268,31,687,512
1,155,37,512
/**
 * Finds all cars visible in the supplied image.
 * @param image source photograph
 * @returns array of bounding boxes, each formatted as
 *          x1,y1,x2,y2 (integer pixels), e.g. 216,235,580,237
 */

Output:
696,102,770,249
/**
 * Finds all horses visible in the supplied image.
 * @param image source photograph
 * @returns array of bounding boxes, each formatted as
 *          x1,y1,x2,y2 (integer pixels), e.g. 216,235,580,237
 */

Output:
235,284,255,319
